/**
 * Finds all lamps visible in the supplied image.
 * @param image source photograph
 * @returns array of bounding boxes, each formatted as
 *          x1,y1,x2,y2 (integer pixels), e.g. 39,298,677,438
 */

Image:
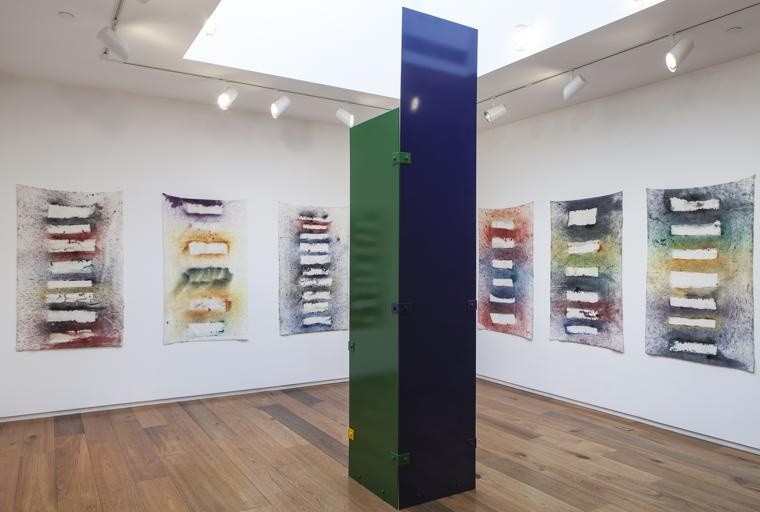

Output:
271,97,290,119
97,27,134,61
667,38,696,71
336,108,355,129
563,75,587,100
216,87,239,110
483,104,505,123
